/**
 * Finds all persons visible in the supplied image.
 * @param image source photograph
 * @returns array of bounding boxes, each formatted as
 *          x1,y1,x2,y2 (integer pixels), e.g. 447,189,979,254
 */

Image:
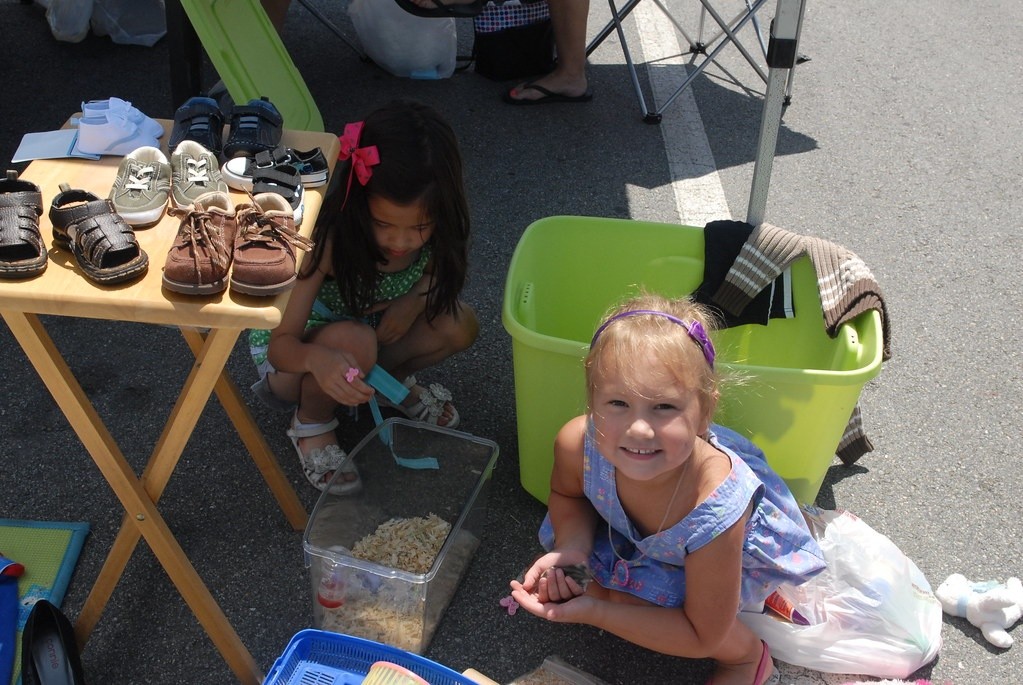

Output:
511,296,829,685
392,0,592,101
250,102,479,494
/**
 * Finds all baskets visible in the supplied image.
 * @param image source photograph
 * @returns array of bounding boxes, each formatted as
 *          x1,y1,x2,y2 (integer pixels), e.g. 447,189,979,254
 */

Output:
259,628,477,685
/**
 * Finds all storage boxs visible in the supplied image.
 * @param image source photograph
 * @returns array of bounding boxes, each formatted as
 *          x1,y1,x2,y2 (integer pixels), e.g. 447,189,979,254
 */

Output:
263,629,479,685
300,417,501,656
501,216,883,505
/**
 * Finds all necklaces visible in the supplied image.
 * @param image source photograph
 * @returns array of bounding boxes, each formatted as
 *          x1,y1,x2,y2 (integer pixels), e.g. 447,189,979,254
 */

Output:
608,465,684,561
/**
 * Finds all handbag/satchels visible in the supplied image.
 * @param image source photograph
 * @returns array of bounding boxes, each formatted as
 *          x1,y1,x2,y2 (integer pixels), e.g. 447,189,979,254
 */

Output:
453,0,557,81
347,0,457,79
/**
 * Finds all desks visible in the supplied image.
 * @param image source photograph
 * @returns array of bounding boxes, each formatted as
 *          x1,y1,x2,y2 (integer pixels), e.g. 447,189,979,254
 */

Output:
0,111,339,685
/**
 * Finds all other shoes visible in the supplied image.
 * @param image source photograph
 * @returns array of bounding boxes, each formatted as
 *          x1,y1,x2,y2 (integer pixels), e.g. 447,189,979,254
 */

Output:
21,600,85,685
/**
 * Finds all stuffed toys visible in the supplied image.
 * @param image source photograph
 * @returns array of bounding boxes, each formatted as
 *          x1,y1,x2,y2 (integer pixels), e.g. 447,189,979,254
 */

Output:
937,574,1023,648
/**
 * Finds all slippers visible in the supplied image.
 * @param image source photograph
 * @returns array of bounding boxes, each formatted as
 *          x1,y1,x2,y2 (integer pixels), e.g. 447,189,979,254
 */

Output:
508,80,593,103
396,0,482,18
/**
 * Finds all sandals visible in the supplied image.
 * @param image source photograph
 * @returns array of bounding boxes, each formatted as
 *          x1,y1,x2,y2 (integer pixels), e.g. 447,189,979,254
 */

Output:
0,170,47,277
48,184,148,284
706,638,780,685
375,374,460,429
286,406,360,496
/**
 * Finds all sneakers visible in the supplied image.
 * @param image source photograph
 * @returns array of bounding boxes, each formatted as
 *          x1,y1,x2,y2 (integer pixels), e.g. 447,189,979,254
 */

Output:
70,112,160,156
80,97,164,138
220,145,330,191
251,165,306,226
167,97,225,161
230,192,315,296
108,146,171,225
169,140,228,211
222,95,282,157
161,190,236,296
